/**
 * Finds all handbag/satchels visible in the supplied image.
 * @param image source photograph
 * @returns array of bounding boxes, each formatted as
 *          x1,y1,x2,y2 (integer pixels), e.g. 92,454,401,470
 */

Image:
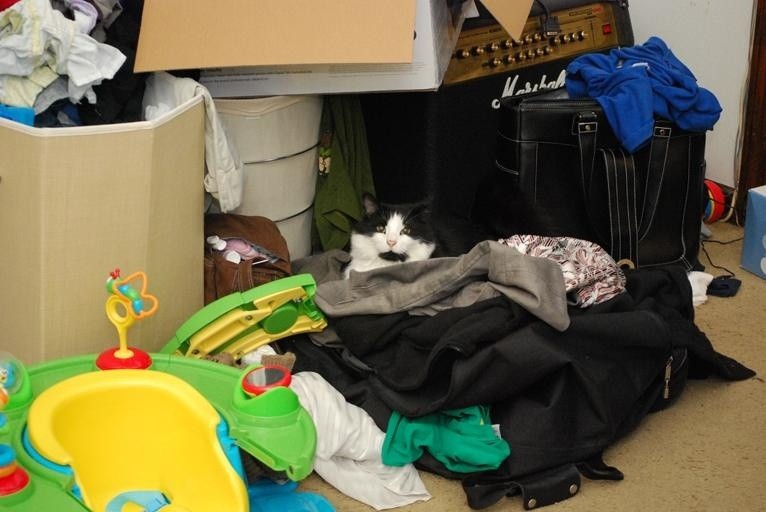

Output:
204,213,292,307
496,86,705,270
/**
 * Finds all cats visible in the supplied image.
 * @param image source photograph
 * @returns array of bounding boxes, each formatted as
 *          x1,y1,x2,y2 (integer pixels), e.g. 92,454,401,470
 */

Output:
344,191,458,282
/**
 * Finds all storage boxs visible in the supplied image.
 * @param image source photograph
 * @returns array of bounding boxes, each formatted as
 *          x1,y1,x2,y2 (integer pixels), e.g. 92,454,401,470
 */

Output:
132,0,537,99
